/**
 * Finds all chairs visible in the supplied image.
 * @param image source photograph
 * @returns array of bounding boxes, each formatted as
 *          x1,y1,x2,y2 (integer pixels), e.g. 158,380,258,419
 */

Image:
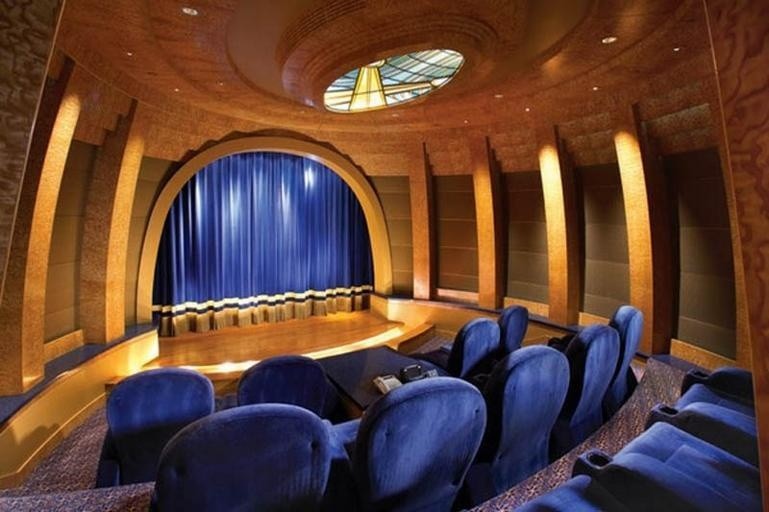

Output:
149,403,350,512
645,366,759,466
95,366,215,489
409,304,645,512
573,421,762,512
228,354,339,419
327,376,488,511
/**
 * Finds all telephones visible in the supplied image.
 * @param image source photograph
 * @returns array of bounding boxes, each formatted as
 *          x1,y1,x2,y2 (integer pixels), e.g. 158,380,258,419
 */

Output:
373,375,402,395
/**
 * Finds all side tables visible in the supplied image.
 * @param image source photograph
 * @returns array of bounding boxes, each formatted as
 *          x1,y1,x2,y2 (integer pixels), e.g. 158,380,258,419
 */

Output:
318,344,441,422
